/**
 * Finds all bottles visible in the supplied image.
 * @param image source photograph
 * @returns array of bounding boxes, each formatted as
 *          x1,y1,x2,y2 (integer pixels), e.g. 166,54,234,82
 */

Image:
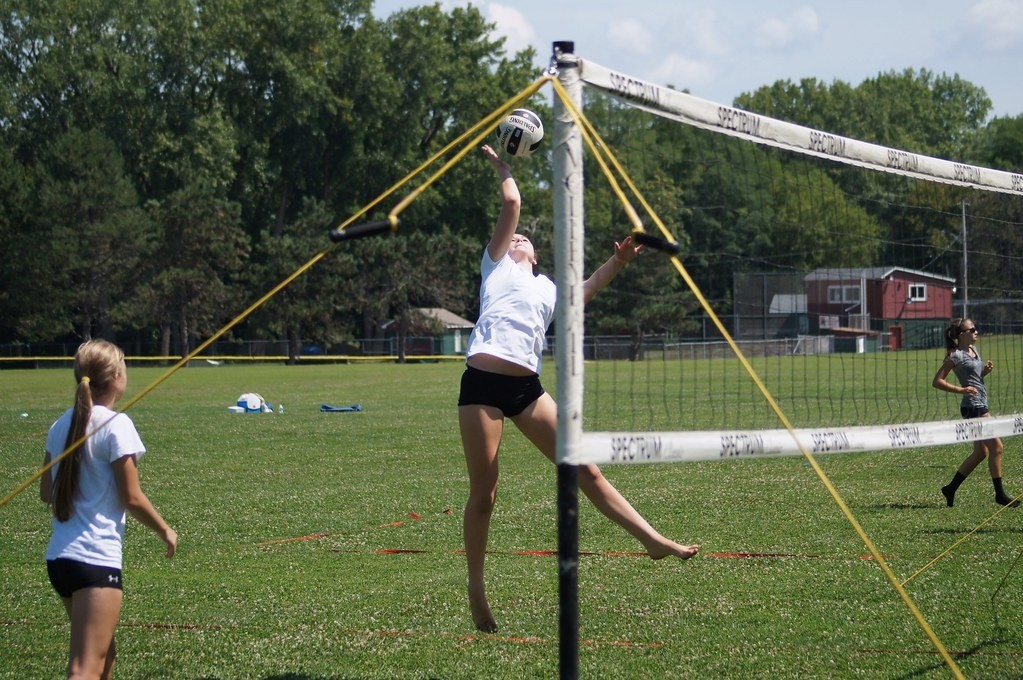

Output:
278,404,283,413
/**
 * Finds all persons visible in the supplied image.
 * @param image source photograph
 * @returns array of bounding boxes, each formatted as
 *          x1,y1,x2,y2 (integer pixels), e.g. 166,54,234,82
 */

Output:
930,316,1022,509
39,338,179,680
456,141,703,634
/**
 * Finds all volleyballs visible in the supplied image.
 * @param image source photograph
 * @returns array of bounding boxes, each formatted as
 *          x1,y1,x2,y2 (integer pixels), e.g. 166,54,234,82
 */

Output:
496,108,544,157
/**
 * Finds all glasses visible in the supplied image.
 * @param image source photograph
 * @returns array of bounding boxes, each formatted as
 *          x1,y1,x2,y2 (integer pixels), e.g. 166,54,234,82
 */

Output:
963,327,978,333
